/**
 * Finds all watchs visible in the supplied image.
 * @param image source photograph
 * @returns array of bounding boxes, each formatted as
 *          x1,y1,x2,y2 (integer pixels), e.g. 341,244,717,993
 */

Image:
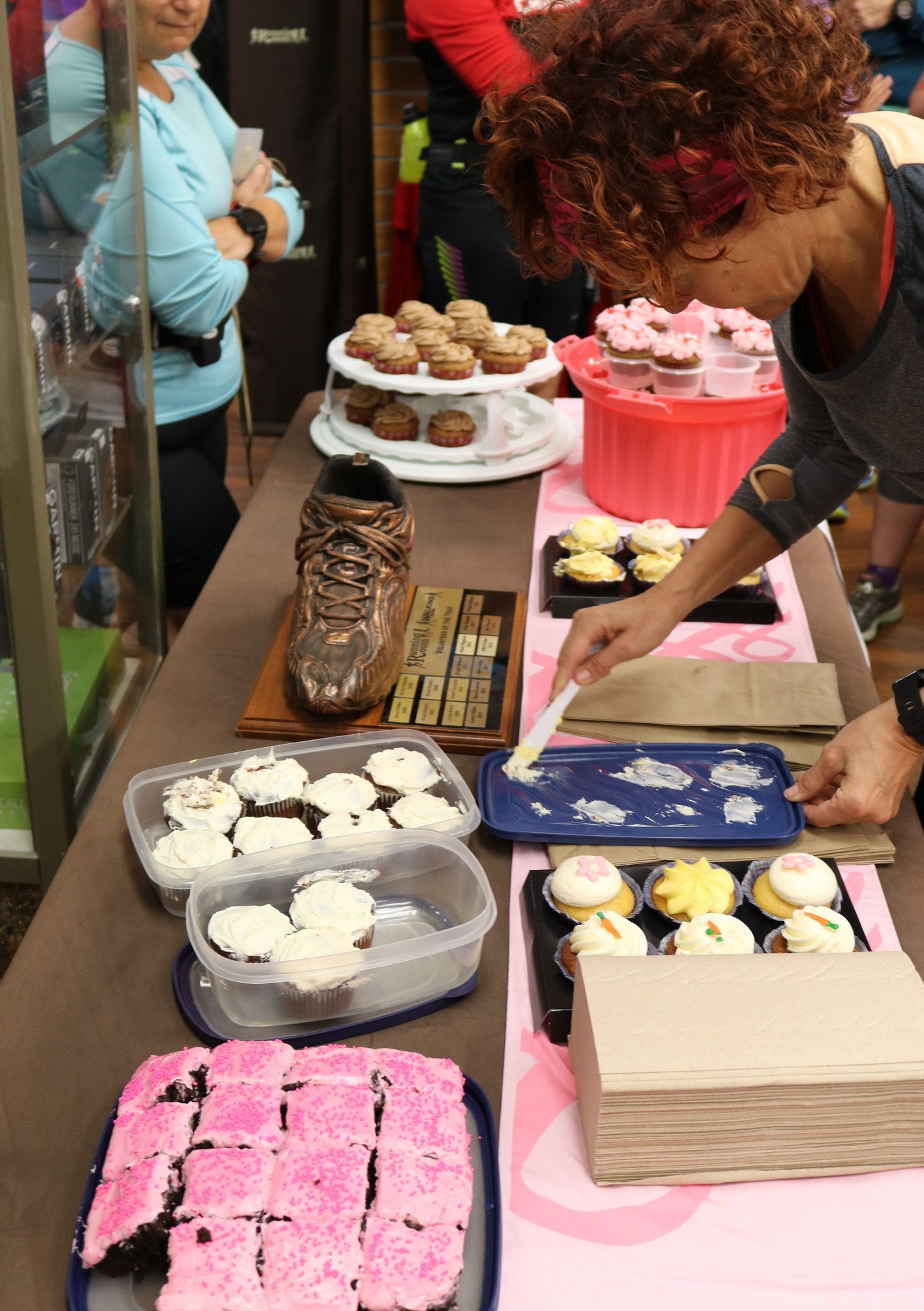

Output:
890,668,924,744
227,208,267,264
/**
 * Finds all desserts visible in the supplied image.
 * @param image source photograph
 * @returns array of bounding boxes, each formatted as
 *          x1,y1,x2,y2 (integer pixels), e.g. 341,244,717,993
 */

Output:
545,851,859,977
344,298,548,448
81,1038,472,1309
207,882,379,1018
153,746,462,914
595,296,778,387
549,515,769,606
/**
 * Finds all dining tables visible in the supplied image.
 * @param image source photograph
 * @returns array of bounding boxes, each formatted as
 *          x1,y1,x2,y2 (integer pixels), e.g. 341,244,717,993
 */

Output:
0,391,924,1311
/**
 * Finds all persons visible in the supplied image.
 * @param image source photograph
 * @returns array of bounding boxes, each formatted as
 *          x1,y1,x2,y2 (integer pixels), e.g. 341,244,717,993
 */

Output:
833,0,923,117
32,0,314,646
401,1,596,351
473,0,924,832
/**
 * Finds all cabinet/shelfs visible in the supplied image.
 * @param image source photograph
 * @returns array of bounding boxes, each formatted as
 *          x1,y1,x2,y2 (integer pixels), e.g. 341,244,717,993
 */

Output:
0,0,170,981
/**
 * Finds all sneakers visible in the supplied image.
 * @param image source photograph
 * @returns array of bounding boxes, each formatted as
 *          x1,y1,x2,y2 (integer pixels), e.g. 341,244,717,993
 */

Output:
848,571,905,642
283,452,417,723
